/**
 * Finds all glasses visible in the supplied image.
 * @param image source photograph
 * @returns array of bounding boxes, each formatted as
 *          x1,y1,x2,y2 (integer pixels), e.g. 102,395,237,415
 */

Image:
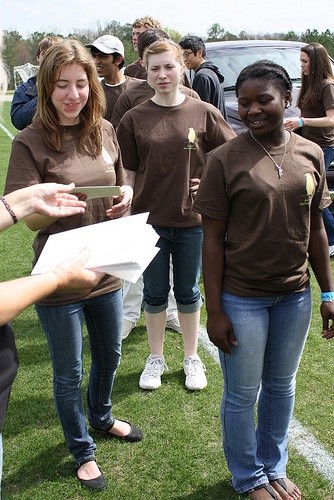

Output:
183,52,194,58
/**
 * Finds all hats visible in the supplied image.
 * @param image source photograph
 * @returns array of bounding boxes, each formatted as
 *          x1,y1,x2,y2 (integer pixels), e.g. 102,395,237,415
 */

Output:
83,35,125,69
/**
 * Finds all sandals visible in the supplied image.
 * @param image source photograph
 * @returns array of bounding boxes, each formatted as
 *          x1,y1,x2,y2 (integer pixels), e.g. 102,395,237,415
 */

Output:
243,483,282,500
269,478,302,500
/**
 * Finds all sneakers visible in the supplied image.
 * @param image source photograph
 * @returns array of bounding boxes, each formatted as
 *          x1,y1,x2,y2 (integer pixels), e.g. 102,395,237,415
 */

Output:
121,319,136,340
138,353,169,389
165,316,182,335
182,355,208,390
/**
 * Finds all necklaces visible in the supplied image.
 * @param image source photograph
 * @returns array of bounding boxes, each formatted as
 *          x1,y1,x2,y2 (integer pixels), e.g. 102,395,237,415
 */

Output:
249,129,287,178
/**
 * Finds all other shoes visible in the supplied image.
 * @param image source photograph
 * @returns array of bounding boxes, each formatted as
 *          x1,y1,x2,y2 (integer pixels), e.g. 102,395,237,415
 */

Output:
90,417,143,442
74,459,106,491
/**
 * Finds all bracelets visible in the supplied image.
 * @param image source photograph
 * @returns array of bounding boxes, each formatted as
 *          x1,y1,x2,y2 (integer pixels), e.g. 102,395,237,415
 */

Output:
298,117,304,128
1,196,18,225
321,292,334,302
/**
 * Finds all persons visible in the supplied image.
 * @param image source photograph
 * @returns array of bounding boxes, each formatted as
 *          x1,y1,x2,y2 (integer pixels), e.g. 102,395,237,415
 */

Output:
285,43,334,257
124,17,191,90
4,40,144,492
84,35,146,121
192,60,334,500
116,41,237,390
111,28,202,340
179,36,228,123
0,182,106,500
10,36,62,130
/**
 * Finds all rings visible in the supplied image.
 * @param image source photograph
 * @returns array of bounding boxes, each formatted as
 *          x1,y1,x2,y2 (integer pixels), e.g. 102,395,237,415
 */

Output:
58,205,61,210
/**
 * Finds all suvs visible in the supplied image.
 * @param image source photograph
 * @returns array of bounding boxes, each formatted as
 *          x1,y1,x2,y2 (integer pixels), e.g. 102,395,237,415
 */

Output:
181,39,334,190
